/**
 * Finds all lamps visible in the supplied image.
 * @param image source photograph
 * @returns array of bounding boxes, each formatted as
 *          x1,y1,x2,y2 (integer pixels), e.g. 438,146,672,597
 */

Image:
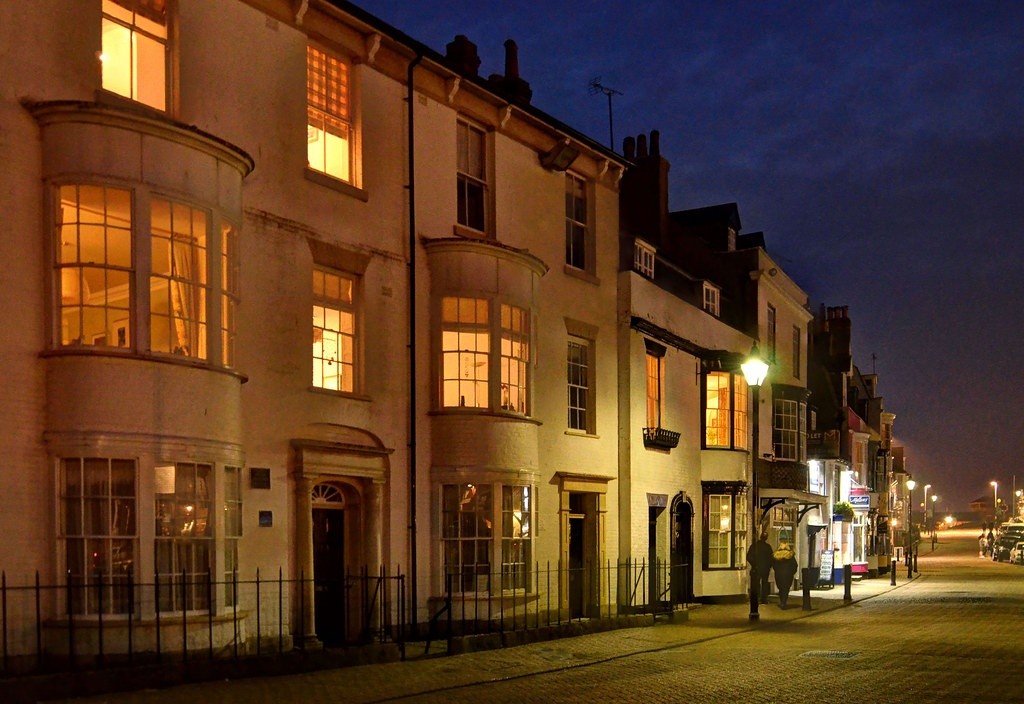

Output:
541,141,581,172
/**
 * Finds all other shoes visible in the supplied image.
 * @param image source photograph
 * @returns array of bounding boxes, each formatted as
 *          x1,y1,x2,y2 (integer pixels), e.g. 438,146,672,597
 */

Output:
780,603,787,610
761,601,768,604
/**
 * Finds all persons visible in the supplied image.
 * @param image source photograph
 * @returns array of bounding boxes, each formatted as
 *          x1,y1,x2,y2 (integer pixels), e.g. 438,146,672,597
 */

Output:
773,542,798,610
978,519,994,558
746,530,774,606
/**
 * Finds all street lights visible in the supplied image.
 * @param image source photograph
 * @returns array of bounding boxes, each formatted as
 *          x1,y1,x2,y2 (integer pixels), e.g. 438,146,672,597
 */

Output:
991,481,997,525
924,484,932,524
930,493,938,536
904,474,916,560
741,340,773,624
